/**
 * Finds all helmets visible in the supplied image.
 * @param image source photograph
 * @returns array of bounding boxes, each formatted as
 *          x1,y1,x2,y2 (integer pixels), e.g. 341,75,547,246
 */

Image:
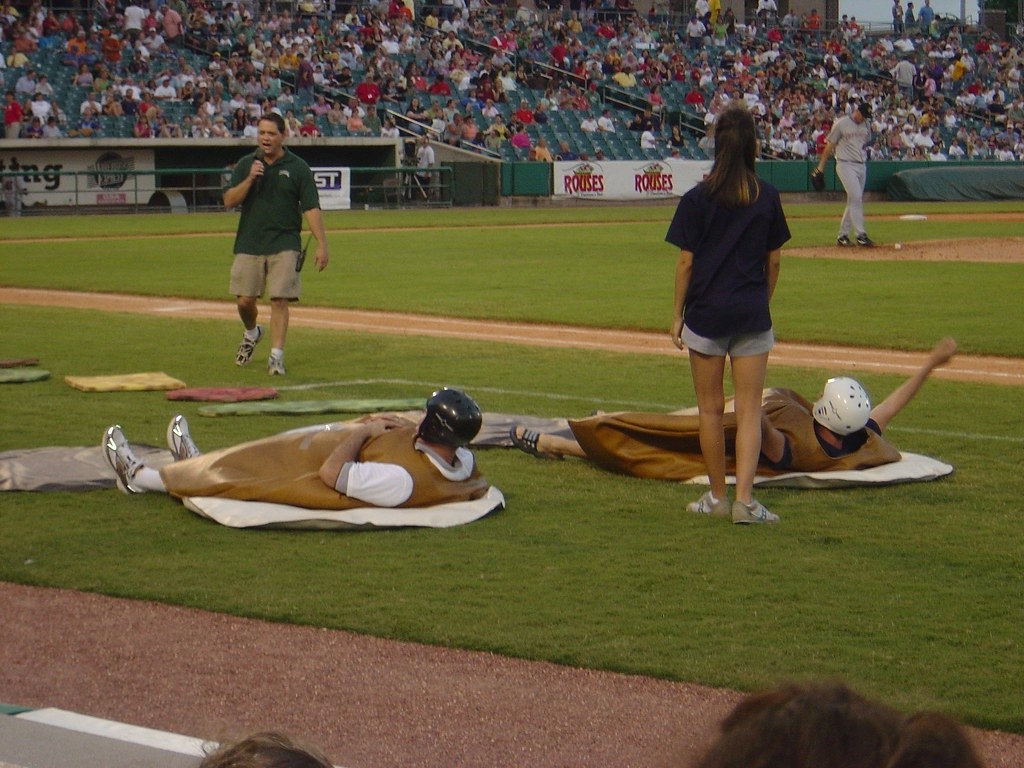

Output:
812,377,870,435
418,388,481,445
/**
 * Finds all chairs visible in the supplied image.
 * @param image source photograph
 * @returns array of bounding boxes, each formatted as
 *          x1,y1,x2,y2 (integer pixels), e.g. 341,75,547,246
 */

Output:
0,0,1024,162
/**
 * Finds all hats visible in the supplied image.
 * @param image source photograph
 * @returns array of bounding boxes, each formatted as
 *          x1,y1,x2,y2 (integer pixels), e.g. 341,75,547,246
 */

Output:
858,103,872,118
366,73,373,78
214,52,220,56
265,42,271,48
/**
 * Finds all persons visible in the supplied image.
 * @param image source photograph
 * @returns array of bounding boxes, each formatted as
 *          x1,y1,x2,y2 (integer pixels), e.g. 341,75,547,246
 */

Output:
0,0,1024,161
665,108,792,524
811,103,875,247
415,135,434,198
224,111,328,374
101,387,482,509
510,337,956,472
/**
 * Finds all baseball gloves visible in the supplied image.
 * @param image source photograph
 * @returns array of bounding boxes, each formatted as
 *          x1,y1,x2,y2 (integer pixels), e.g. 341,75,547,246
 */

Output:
810,167,825,192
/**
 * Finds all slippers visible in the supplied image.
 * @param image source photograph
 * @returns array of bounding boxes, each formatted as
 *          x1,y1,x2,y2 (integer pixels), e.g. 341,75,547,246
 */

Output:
510,426,564,460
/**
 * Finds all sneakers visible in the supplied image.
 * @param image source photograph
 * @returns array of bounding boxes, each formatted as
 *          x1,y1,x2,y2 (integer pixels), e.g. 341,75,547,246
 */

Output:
167,414,199,462
102,425,144,495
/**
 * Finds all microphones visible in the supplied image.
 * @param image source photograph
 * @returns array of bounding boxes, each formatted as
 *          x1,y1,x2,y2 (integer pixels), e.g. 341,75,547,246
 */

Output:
253,150,266,194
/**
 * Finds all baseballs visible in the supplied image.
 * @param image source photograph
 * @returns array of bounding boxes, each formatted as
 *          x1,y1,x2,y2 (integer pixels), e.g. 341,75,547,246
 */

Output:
895,243,901,250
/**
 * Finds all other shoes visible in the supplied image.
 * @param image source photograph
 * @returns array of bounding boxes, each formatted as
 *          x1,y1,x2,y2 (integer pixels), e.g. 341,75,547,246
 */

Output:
267,354,285,375
732,500,779,524
857,238,880,248
236,325,264,366
838,236,855,247
686,491,730,515
